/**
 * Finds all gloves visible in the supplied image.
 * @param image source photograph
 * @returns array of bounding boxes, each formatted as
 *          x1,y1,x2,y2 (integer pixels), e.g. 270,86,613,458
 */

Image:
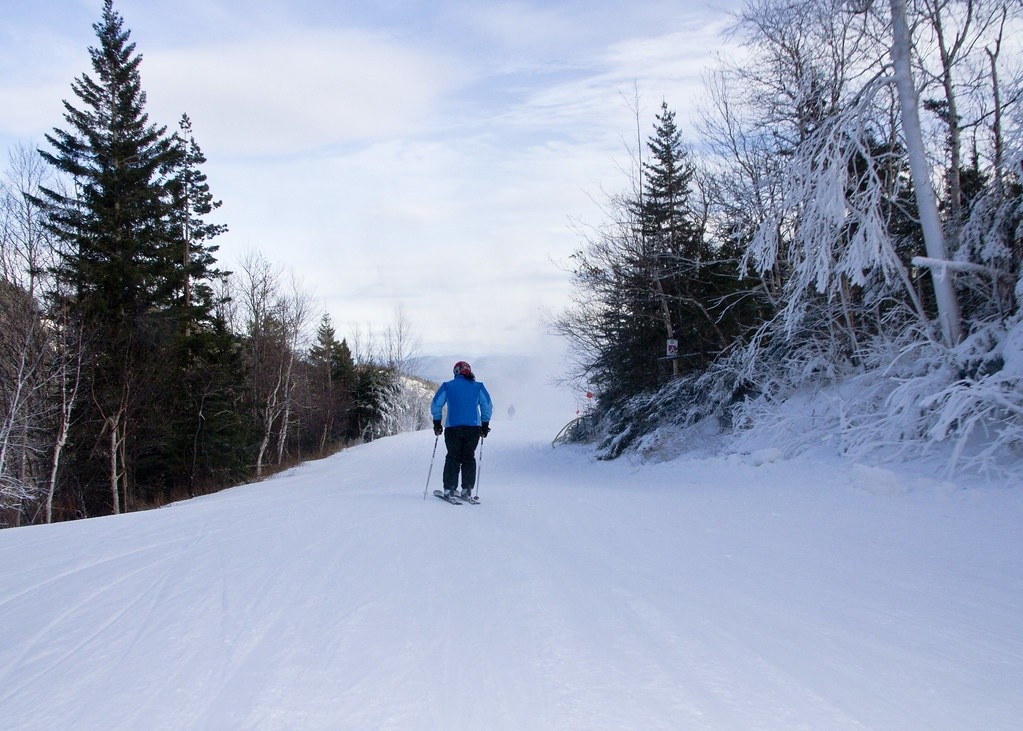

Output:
433,420,443,435
479,422,489,438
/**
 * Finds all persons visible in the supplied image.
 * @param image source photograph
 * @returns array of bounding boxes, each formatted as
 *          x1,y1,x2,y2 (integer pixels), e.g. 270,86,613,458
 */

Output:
508,404,515,420
430,360,494,498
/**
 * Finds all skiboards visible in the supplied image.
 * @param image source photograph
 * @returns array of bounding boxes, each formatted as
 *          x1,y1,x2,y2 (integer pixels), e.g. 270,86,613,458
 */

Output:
433,489,482,505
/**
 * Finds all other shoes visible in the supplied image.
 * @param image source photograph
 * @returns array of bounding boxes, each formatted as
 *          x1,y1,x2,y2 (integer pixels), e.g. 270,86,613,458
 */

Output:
461,489,471,499
444,489,453,499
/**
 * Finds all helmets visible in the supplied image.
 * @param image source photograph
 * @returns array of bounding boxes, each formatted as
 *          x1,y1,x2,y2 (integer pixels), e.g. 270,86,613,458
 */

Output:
453,362,472,375
